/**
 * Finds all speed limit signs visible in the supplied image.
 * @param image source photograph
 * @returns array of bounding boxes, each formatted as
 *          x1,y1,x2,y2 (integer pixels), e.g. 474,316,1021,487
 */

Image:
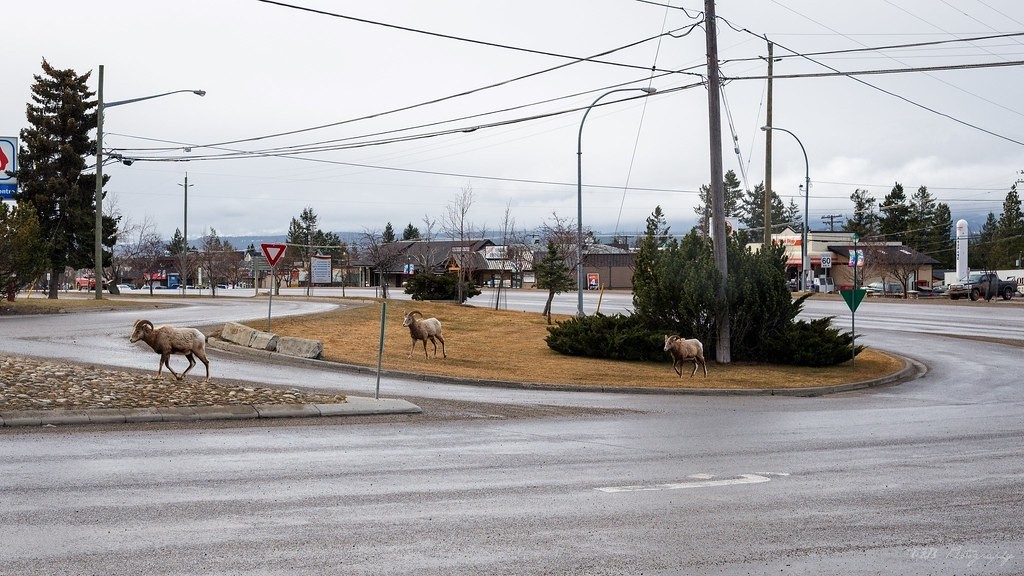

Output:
821,253,831,268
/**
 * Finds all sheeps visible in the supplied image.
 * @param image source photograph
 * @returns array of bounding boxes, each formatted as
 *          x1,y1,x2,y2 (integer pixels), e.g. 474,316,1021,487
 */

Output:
401,310,447,359
663,335,707,379
129,319,210,382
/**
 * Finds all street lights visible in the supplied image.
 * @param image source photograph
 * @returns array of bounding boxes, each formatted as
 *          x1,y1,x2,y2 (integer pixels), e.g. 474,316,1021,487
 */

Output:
177,172,194,289
760,126,810,292
96,64,206,300
577,88,657,316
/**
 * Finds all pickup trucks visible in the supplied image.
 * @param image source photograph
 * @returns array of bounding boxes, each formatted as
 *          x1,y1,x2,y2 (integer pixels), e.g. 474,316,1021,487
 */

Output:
949,271,1018,301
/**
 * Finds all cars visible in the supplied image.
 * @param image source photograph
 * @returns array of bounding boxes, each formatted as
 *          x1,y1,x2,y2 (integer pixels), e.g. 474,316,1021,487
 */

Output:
859,281,904,298
75,273,108,291
